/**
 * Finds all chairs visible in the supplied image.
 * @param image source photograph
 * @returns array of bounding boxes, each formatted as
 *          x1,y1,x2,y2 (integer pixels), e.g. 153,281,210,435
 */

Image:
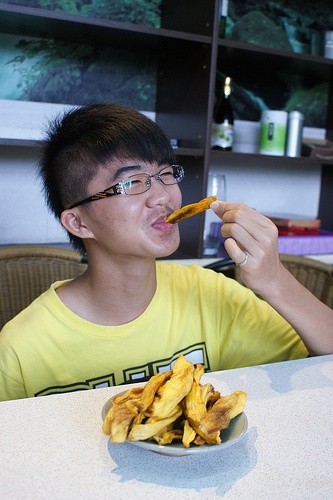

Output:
202,254,333,359
0,247,88,332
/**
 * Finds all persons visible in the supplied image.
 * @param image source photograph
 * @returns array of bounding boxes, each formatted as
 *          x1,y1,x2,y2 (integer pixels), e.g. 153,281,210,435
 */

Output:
0,104,333,401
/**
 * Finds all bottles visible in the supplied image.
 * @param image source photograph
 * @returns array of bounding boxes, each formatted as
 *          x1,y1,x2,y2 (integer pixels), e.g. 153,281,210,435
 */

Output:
210,77,235,152
259,110,288,157
285,110,305,158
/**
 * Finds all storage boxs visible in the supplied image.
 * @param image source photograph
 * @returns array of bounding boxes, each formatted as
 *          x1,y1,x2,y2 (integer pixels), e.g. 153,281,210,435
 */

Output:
263,213,321,237
302,139,333,159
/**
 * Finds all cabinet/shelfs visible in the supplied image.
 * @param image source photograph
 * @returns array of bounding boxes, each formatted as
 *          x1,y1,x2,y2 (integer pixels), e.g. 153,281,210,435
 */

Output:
0,0,333,260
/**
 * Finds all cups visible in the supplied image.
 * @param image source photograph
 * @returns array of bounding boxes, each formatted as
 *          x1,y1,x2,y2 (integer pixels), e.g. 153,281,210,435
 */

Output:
233,119,260,154
202,172,229,257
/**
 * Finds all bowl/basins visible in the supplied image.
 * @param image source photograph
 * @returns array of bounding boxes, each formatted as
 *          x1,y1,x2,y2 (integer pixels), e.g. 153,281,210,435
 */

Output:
101,387,249,457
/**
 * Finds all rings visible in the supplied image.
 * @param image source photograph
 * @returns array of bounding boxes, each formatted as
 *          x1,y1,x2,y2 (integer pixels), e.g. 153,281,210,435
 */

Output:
235,251,250,267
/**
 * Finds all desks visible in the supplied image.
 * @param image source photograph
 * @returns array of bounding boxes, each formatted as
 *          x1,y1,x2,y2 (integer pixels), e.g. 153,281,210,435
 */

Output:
0,354,333,500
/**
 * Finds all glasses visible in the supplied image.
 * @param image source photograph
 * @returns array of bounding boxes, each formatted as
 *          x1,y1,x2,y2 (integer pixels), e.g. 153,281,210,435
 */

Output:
67,164,185,209
